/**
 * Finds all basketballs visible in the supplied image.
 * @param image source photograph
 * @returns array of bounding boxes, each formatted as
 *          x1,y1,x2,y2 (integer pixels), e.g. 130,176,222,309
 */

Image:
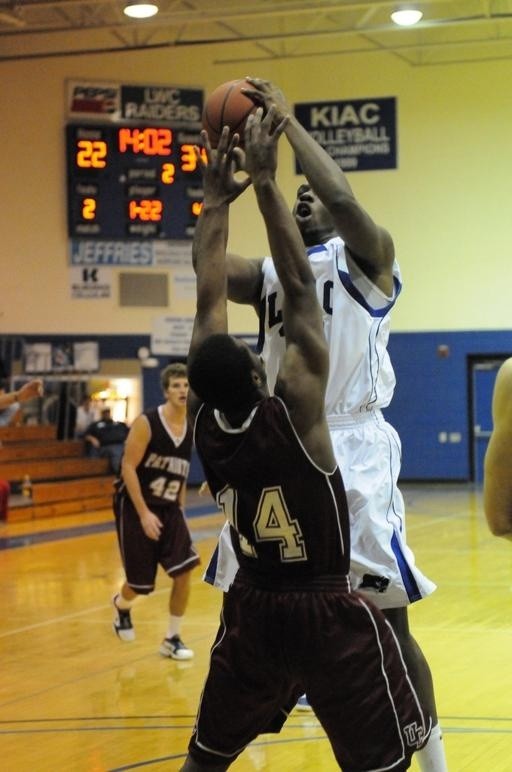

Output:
203,77,275,150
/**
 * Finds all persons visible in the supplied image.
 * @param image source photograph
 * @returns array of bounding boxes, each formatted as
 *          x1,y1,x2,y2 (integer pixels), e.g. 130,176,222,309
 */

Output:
482,355,512,543
1,366,99,442
81,406,131,474
112,363,196,660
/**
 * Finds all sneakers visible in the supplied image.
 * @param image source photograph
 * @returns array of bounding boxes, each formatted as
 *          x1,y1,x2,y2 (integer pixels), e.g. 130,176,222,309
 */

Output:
110,593,136,641
157,633,197,662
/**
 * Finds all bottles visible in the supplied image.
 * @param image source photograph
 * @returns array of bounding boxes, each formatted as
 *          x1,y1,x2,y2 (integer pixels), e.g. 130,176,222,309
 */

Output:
20,474,31,497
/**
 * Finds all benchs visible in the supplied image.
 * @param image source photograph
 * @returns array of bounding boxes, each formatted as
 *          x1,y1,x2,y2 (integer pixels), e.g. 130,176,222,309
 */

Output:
0,425,129,518
